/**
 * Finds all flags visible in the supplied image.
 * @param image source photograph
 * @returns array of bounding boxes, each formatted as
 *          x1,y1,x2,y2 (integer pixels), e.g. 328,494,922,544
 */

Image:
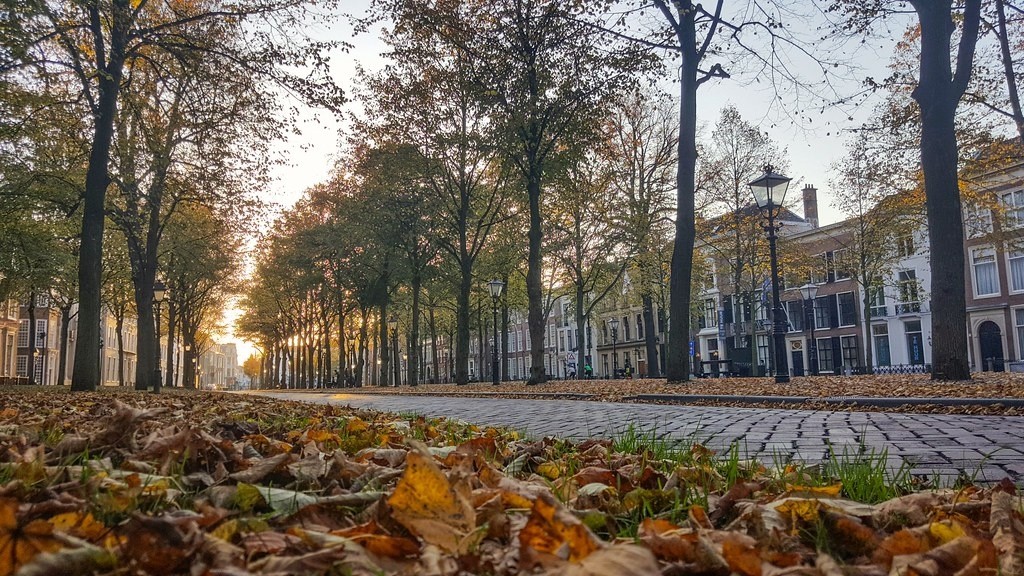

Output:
761,277,772,303
622,271,634,295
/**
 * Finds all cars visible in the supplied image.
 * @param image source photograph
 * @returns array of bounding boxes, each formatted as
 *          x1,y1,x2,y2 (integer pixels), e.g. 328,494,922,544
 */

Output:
206,384,216,390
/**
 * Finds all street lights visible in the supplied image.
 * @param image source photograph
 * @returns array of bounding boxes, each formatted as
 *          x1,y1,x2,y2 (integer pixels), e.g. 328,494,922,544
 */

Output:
348,336,355,387
486,276,506,384
798,277,821,375
153,279,166,394
607,317,620,379
402,354,407,386
254,355,303,389
377,358,380,386
191,355,204,390
185,343,192,388
322,348,327,389
388,316,399,385
748,162,793,382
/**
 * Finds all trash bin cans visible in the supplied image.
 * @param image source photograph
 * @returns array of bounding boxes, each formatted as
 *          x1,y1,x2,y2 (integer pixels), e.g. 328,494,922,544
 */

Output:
993,357,1005,372
637,358,647,374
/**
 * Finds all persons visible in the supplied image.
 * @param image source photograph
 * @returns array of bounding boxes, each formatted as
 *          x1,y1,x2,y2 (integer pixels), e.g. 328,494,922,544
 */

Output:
624,366,629,379
568,364,592,380
